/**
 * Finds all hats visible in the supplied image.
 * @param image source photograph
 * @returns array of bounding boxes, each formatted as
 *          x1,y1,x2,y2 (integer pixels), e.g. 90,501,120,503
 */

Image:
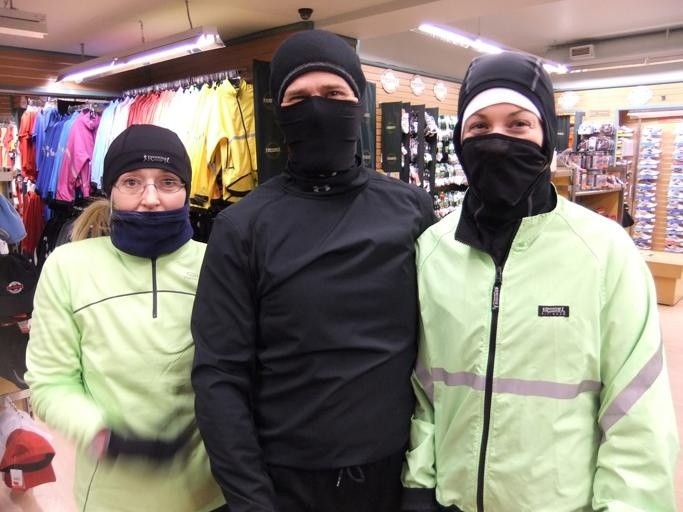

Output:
0,429,57,489
269,28,367,110
103,123,192,198
0,254,38,317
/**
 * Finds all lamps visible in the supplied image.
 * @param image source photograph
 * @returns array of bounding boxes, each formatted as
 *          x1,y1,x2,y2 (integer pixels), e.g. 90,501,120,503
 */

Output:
56,1,226,85
0,0,48,39
413,21,569,75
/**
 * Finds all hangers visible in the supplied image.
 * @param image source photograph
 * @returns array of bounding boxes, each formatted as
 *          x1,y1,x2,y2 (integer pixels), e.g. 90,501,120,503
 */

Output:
25,97,95,117
121,70,238,98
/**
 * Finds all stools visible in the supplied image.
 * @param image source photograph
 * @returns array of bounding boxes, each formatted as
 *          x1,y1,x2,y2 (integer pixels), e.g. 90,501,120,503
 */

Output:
637,250,683,305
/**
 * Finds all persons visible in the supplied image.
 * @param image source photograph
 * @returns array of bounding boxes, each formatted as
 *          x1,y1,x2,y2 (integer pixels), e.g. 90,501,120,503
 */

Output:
21,122,254,510
398,49,672,511
190,31,444,509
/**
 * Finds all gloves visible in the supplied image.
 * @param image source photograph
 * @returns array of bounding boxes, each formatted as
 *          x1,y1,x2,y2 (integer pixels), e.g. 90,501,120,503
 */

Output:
107,433,175,465
